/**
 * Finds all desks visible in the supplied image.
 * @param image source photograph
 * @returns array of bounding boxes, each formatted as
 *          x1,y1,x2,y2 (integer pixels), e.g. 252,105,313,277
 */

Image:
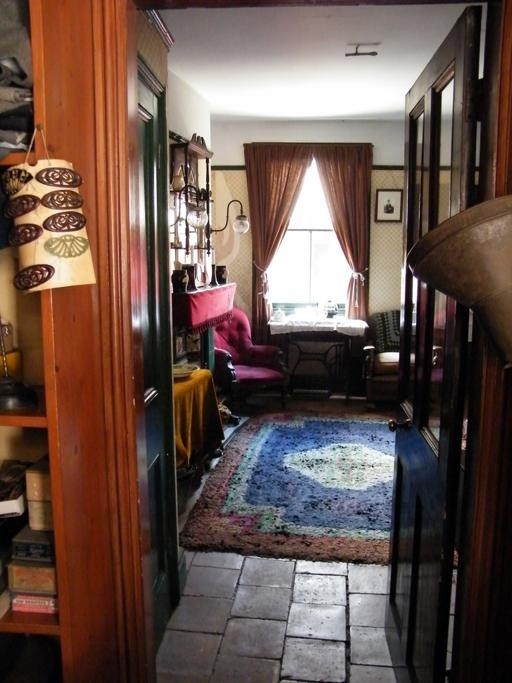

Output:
267,315,370,398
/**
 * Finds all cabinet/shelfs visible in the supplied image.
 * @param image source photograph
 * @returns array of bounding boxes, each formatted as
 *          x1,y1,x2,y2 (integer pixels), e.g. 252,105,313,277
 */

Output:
0,7,116,683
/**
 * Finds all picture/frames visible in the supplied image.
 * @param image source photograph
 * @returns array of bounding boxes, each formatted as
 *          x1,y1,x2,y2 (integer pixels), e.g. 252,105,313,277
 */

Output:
374,185,403,223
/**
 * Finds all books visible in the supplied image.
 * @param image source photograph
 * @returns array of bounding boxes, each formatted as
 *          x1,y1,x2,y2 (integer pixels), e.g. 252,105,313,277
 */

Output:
0,459,58,621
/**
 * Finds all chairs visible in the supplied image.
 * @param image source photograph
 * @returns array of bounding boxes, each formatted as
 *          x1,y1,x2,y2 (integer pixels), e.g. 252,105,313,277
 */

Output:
360,307,444,406
213,306,290,417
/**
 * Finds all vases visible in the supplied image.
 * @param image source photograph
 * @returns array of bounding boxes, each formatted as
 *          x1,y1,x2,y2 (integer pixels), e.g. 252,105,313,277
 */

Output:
171,263,197,293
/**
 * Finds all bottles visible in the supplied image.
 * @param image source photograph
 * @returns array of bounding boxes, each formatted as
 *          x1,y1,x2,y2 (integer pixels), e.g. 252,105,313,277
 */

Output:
217,266,228,284
173,270,188,292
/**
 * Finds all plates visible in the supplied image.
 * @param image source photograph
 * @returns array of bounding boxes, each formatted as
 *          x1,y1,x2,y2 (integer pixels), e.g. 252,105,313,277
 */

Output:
173,364,198,378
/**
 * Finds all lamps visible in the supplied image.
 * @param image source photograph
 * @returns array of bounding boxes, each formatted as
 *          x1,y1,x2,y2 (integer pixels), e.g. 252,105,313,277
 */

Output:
169,183,250,255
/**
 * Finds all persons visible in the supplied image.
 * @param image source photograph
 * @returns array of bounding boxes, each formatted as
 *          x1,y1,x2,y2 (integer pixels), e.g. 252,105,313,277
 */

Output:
386,199,394,213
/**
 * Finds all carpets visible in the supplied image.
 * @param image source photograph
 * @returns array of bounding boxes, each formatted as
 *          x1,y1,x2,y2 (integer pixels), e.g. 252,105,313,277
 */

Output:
180,402,466,564
